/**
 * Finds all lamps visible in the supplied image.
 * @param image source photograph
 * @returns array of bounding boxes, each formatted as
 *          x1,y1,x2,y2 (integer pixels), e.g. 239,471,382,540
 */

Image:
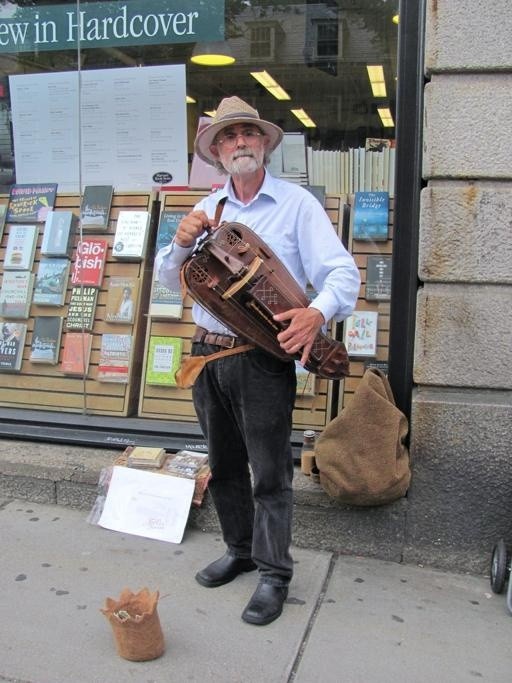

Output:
189,39,236,66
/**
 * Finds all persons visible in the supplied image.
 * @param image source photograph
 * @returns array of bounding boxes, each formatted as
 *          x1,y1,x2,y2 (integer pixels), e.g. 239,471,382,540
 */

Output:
154,94,364,627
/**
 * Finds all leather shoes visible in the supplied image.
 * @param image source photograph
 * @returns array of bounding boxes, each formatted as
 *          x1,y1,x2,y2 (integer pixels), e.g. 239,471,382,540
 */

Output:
241,578,288,625
194,550,258,588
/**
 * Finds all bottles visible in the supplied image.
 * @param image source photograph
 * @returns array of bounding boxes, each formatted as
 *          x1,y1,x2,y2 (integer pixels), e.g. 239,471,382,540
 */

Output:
299,427,317,478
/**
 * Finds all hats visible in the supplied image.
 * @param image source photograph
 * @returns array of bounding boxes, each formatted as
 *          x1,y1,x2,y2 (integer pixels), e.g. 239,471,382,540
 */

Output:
193,95,284,168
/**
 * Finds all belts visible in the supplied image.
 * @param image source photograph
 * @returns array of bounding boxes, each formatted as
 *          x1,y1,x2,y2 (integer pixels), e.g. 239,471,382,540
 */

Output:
193,326,250,351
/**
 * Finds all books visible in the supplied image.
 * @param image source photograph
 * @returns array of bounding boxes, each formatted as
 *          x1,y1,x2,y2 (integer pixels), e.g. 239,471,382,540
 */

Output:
97,333,131,385
343,311,378,358
146,337,183,386
149,211,192,320
79,185,114,230
1,183,78,372
353,191,389,242
66,286,99,331
71,240,109,289
294,359,315,397
112,211,151,259
128,443,210,480
306,291,328,336
190,118,396,205
365,254,393,302
104,277,140,324
62,333,93,377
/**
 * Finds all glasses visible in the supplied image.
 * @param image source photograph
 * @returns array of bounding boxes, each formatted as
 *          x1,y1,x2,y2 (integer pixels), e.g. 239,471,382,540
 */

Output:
218,129,263,150
